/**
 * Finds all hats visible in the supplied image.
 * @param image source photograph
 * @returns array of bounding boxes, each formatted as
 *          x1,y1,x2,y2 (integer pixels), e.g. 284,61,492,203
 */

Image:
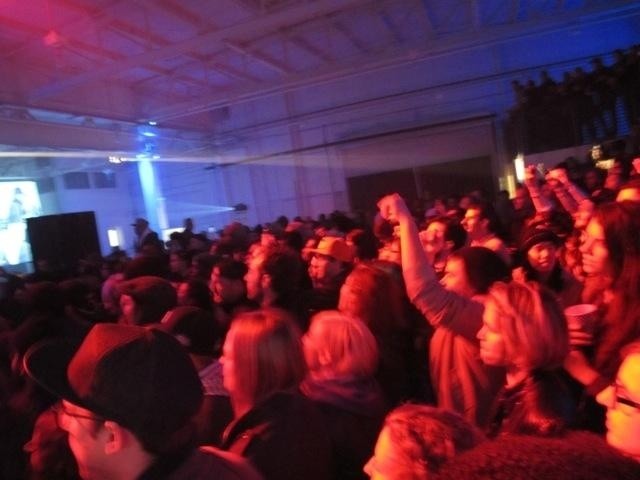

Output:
144,307,216,349
212,259,244,278
116,277,178,307
518,228,560,251
308,238,352,263
25,323,205,434
130,218,148,227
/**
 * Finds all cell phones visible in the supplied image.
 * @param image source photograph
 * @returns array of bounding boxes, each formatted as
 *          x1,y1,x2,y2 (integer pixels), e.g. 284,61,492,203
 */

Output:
550,169,560,179
536,163,548,176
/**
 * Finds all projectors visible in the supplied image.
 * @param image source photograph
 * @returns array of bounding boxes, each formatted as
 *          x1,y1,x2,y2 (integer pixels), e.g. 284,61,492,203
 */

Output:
233,203,247,212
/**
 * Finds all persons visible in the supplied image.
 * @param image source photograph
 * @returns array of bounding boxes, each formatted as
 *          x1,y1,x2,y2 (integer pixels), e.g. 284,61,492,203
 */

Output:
359,221,400,262
477,282,605,434
24,324,261,480
537,203,640,433
523,147,640,196
24,407,76,480
301,266,390,405
517,229,584,318
173,279,209,310
313,236,356,302
301,311,396,414
116,277,177,326
571,197,598,228
145,305,233,431
418,187,509,214
311,213,359,234
182,219,196,237
426,218,468,258
595,341,640,462
440,436,635,480
0,269,23,415
378,193,512,428
461,202,505,253
513,183,532,218
210,260,261,356
25,256,119,321
244,243,320,329
167,231,218,277
218,312,335,480
561,235,585,306
111,218,164,274
362,404,489,479
213,216,310,258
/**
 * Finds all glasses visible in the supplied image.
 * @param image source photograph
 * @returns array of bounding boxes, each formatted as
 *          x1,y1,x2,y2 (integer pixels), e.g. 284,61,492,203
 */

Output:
610,379,640,411
53,401,112,435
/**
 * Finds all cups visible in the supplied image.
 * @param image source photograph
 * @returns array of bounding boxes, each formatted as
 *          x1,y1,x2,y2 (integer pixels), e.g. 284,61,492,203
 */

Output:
564,304,600,332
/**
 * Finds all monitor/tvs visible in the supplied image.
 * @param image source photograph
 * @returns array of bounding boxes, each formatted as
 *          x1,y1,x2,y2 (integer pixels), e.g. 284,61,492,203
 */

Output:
25,211,103,277
